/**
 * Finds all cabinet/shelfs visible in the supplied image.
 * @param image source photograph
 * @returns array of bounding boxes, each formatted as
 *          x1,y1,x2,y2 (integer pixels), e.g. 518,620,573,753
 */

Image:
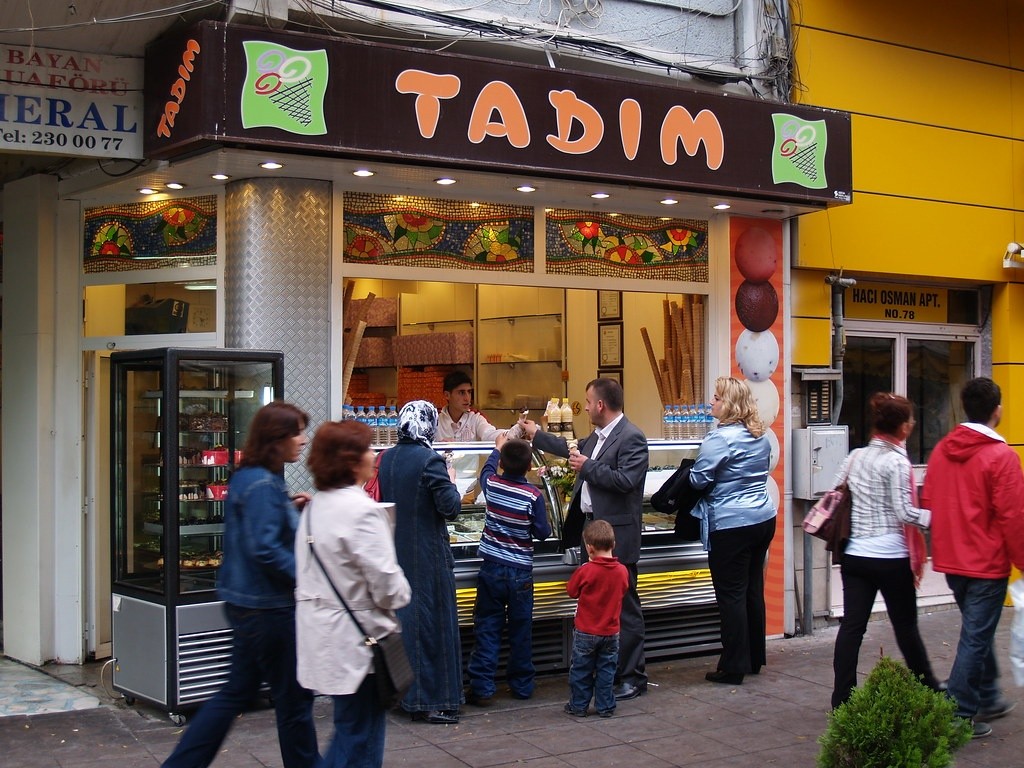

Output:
403,319,474,410
480,312,562,411
109,347,286,729
345,326,397,410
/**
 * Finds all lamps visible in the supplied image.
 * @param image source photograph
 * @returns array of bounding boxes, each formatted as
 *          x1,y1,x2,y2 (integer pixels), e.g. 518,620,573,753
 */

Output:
1002,242,1024,270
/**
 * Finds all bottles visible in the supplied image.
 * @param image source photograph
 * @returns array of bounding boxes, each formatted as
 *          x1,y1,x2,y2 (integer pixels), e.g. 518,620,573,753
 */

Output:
342,404,399,447
662,404,716,441
547,397,574,440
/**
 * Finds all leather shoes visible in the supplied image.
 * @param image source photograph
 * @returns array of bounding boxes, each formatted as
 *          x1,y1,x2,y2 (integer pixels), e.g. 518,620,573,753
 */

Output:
612,681,647,700
412,710,459,722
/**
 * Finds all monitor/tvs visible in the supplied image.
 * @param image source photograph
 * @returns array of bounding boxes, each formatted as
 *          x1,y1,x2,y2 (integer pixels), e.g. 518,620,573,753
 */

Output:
125,297,190,336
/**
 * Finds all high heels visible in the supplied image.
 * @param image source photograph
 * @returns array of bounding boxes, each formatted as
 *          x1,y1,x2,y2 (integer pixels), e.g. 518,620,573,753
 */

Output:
748,664,762,674
706,671,743,685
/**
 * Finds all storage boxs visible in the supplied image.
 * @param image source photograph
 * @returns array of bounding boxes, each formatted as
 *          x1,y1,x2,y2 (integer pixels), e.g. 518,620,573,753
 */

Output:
344,331,474,408
511,394,543,409
487,352,514,362
344,296,397,326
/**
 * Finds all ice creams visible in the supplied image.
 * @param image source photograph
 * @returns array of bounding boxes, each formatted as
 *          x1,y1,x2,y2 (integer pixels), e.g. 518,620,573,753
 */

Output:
519,404,530,423
566,438,579,455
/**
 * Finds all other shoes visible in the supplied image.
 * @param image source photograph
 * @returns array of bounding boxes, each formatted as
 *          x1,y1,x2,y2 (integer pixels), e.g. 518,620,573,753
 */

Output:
936,679,953,697
970,723,992,738
974,697,1017,720
470,692,495,703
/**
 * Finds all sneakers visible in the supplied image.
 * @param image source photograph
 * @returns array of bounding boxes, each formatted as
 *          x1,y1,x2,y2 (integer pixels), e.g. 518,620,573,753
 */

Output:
601,709,614,717
564,702,586,717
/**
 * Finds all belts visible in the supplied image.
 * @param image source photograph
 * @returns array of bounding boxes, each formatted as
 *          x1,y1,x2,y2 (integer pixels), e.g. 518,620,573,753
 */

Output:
585,511,594,521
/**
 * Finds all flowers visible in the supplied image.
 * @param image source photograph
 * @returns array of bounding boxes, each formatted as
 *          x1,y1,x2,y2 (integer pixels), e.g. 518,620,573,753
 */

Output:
536,457,576,502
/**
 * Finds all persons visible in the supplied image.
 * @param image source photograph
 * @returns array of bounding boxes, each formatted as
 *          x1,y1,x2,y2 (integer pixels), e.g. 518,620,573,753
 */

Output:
687,376,778,683
155,403,325,768
563,519,628,718
433,371,531,503
290,418,413,768
917,377,1024,740
375,400,466,726
467,432,554,712
518,378,652,699
829,392,949,711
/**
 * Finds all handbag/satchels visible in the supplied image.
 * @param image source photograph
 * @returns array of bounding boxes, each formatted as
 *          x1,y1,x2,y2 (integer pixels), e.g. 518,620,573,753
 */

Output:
375,632,415,695
801,450,861,540
364,451,389,500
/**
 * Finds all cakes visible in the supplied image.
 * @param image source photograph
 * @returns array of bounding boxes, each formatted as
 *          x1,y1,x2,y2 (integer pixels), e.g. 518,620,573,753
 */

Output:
141,405,244,566
